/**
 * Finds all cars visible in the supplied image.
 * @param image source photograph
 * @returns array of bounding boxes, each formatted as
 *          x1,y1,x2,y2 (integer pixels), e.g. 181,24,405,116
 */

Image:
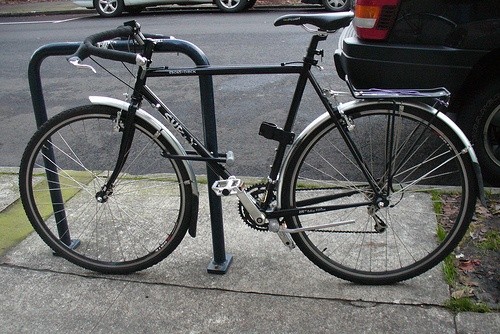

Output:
333,0,500,177
70,0,256,17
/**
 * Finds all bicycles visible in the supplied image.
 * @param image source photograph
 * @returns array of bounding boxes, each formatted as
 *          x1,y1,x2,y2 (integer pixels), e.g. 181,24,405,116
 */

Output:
18,10,488,286
